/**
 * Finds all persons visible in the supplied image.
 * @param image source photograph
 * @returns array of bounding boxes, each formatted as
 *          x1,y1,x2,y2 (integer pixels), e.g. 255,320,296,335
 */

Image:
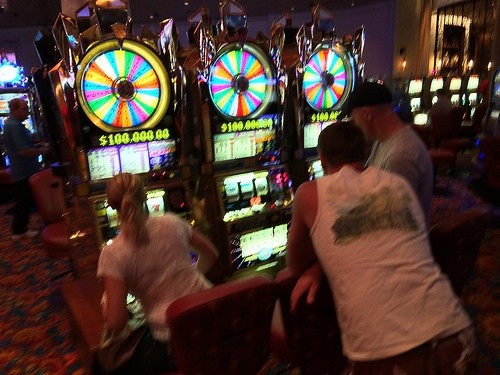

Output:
2,100,54,237
95,173,216,375
285,83,475,375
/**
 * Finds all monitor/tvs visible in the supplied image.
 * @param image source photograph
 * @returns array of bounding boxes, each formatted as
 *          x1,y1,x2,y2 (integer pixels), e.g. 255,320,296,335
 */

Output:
85,115,354,265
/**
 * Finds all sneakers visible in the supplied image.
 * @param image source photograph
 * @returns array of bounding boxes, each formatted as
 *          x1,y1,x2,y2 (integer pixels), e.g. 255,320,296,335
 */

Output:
11,230,39,239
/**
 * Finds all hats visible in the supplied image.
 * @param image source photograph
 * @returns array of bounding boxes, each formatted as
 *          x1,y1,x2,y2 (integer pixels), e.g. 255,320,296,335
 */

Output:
336,82,393,119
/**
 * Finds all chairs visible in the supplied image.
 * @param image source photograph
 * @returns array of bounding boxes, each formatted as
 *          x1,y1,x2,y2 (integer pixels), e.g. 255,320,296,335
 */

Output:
27,102,500,375
